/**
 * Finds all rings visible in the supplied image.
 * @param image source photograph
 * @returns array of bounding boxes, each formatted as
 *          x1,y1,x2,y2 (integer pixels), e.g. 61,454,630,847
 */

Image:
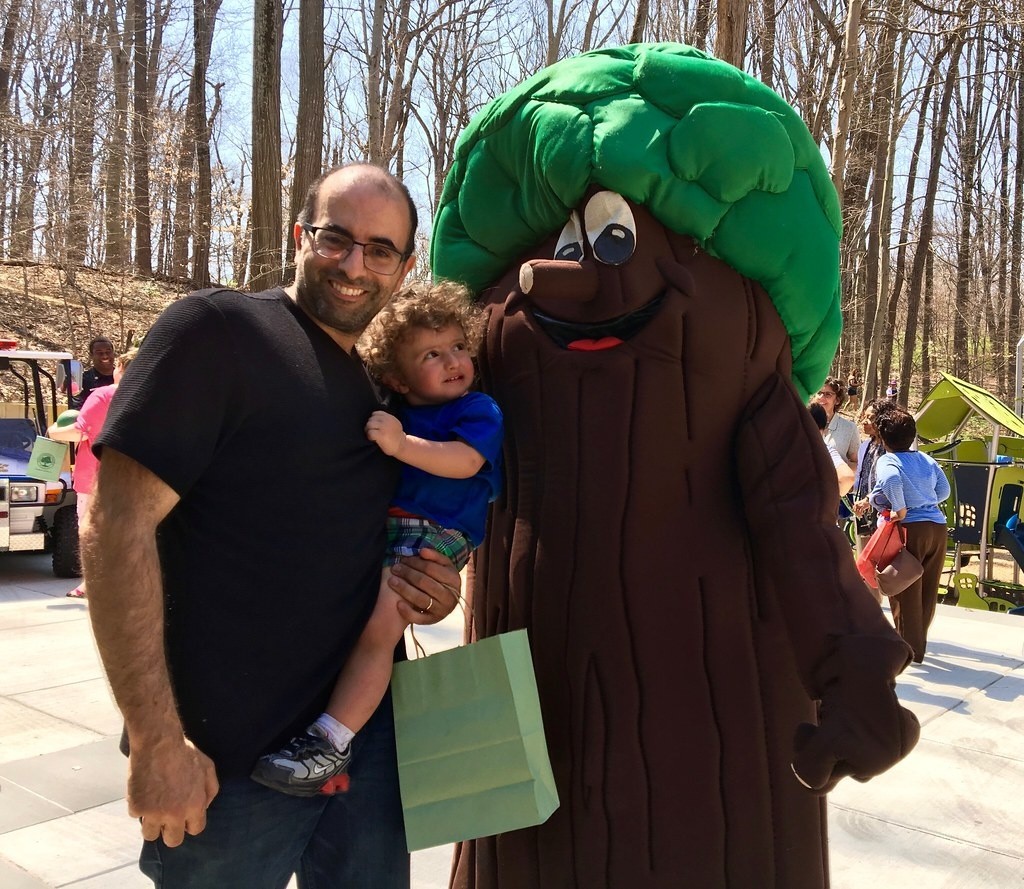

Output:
426,599,433,611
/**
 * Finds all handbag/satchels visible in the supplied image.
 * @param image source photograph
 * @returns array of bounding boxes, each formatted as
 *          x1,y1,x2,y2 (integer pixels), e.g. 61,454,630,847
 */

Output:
856,518,907,588
26,431,68,483
391,585,561,853
874,547,924,596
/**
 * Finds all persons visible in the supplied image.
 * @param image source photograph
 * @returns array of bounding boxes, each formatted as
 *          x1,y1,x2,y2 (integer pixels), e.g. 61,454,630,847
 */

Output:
885,381,898,402
870,410,952,666
807,376,860,531
842,370,863,411
81,337,115,404
249,281,503,796
47,349,140,598
82,163,462,889
853,400,898,604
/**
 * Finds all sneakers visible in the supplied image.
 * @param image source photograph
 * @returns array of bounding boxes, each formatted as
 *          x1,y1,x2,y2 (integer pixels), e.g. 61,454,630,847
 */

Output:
250,724,352,797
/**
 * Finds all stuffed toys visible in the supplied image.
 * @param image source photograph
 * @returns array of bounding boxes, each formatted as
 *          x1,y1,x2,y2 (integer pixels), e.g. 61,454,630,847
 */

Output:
432,41,921,889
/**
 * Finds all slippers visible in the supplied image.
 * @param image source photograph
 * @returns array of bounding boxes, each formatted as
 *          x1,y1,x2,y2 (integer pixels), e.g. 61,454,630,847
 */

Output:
66,582,88,597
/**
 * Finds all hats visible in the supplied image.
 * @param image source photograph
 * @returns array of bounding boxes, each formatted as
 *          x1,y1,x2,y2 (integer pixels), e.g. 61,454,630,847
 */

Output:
891,381,896,385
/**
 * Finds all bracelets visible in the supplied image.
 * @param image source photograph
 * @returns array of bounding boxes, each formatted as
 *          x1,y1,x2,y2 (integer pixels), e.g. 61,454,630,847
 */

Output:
895,393,897,395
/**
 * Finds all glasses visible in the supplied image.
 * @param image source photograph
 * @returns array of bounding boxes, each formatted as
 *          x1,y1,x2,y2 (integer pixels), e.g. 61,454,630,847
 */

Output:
304,222,407,275
816,391,836,398
